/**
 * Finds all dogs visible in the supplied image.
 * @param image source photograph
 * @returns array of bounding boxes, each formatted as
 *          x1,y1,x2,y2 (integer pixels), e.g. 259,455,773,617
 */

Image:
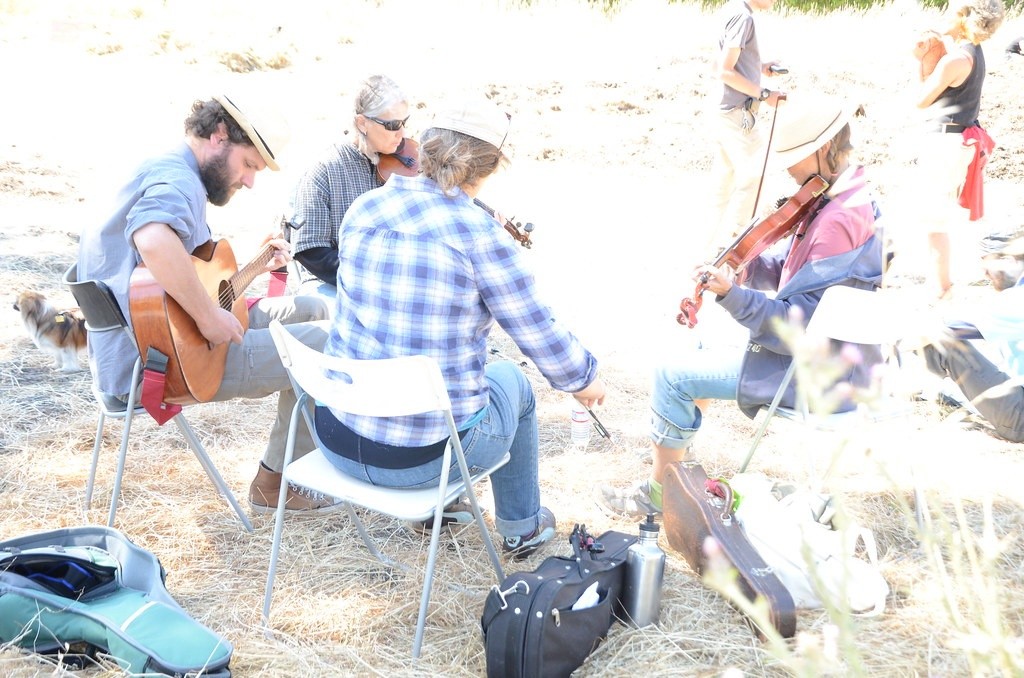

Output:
13,291,88,375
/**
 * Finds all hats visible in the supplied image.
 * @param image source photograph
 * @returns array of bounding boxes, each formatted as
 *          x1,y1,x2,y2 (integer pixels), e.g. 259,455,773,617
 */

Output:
431,97,512,162
213,84,285,172
771,85,861,168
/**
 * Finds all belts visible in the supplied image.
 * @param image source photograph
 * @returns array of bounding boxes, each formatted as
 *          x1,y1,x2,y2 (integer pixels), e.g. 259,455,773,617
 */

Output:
932,119,980,133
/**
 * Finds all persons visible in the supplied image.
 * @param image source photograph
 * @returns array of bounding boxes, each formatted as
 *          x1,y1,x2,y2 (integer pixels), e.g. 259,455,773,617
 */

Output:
597,100,884,523
924,228,1024,443
916,0,1003,302
75,87,347,515
708,0,784,226
290,74,607,563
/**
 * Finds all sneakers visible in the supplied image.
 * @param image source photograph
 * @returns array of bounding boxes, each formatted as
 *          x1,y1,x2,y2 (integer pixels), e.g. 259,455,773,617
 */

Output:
412,501,484,534
592,480,661,523
248,460,339,514
502,506,555,563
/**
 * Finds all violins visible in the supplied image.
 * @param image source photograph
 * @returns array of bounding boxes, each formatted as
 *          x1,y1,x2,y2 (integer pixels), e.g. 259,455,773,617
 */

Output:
677,173,829,331
375,137,538,250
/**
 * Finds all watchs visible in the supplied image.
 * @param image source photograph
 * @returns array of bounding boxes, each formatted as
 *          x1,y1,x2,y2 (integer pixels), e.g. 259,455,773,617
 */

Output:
758,89,770,102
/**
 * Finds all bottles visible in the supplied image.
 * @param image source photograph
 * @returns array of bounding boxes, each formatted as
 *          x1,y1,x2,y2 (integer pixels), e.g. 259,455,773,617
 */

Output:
572,399,590,444
621,513,665,630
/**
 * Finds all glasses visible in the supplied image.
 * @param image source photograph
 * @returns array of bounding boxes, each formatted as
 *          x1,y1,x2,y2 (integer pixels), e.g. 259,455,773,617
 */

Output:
367,116,410,131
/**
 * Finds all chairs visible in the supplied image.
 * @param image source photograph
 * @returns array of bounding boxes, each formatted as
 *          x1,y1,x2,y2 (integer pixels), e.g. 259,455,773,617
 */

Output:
736,285,908,473
62,262,254,533
260,320,511,658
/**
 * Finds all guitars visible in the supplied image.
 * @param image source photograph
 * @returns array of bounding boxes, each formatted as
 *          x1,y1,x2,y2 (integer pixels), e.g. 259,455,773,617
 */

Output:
129,210,309,402
